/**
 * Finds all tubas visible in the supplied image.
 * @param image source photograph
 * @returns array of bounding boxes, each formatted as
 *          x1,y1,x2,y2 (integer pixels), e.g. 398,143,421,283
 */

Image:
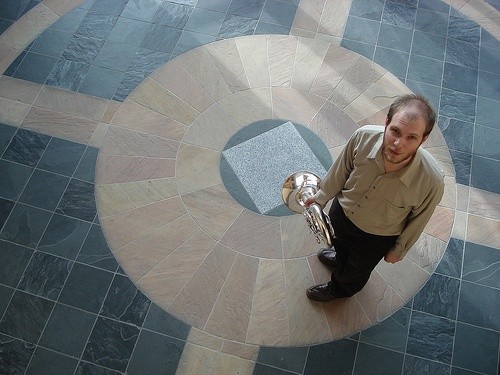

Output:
280,169,337,249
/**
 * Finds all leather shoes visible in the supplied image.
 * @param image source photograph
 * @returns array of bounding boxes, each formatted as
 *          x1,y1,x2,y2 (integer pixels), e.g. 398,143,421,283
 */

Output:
317,247,337,267
306,282,336,301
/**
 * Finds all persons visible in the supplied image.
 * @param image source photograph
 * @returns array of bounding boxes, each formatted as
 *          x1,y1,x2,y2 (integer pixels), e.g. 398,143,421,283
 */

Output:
305,94,445,302
